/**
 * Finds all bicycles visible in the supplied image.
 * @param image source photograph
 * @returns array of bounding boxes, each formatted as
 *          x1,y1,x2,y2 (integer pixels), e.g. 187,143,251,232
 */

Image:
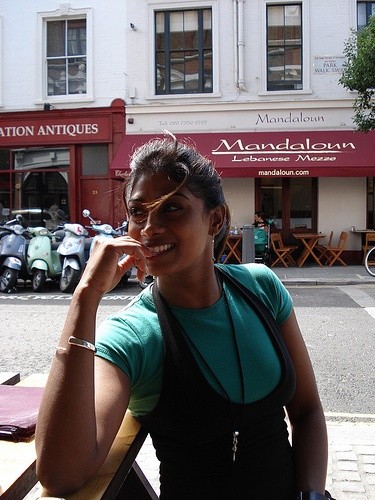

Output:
364,246,375,277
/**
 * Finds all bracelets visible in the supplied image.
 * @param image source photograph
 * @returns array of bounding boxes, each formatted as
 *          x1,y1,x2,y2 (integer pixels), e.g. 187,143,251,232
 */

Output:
298,491,325,500
56,335,98,352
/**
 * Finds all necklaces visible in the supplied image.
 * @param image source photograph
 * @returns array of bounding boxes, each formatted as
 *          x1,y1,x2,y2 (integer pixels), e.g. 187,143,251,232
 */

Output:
161,269,245,461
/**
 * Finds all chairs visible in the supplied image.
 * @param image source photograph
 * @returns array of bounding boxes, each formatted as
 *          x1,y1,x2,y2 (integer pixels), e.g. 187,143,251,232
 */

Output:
269,230,350,268
362,232,375,266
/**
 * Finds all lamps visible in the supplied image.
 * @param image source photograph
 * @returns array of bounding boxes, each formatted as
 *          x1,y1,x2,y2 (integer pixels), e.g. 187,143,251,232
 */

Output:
127,119,133,123
43,103,55,111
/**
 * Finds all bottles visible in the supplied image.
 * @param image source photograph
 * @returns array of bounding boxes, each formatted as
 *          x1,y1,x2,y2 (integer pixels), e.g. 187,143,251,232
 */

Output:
235,225,238,234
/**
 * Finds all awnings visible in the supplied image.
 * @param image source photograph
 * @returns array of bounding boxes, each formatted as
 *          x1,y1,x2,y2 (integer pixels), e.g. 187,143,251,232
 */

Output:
110,130,375,177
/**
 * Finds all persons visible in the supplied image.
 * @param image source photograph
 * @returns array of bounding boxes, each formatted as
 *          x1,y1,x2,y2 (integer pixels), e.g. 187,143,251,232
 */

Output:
251,212,269,228
44,199,65,232
35,138,328,500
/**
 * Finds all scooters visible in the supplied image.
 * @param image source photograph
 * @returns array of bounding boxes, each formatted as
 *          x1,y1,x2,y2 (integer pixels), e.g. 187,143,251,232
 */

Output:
57,219,94,294
82,209,133,294
26,220,66,293
0,213,33,292
138,272,155,289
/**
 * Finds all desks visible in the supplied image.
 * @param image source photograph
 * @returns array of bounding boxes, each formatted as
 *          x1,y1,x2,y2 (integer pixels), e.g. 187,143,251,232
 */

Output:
0,372,158,500
351,230,375,261
223,235,242,263
294,233,326,268
